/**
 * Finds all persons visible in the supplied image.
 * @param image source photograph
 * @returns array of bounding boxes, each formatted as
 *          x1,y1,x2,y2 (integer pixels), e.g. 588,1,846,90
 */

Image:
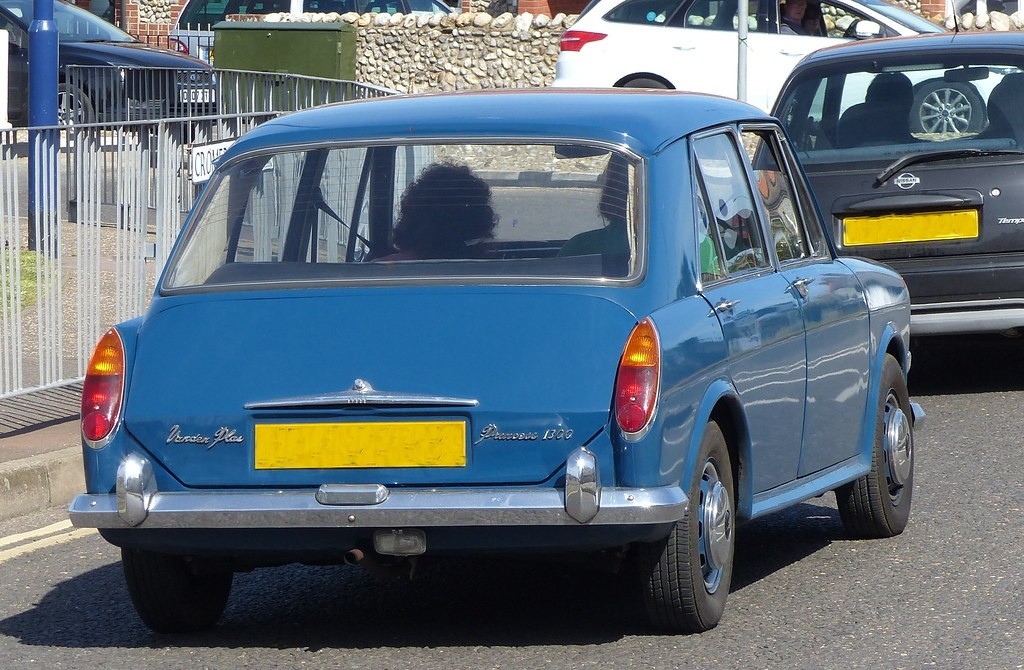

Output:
864,73,928,144
368,160,500,261
780,0,821,36
555,152,721,282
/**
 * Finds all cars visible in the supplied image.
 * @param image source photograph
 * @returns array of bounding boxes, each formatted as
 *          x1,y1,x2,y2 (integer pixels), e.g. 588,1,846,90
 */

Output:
753,31,1023,333
172,0,458,68
0,0,217,141
68,84,927,632
549,0,1024,135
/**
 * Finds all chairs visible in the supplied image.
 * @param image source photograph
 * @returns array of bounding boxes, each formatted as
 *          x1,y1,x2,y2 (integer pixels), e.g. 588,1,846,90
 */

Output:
836,101,912,148
976,72,1024,145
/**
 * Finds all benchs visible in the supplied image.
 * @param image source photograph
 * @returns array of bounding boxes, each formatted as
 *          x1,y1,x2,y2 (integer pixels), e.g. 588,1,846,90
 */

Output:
201,252,633,284
